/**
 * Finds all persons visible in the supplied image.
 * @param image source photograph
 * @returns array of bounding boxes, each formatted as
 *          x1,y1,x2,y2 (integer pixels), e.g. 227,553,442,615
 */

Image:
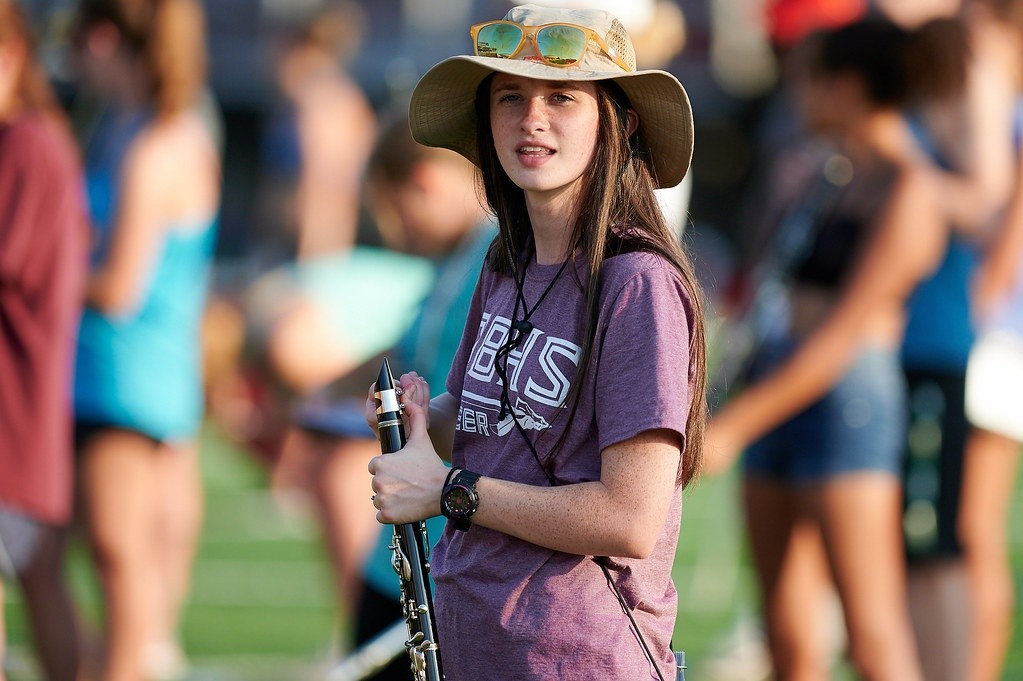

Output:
620,0,1023,681
365,5,707,681
0,0,501,681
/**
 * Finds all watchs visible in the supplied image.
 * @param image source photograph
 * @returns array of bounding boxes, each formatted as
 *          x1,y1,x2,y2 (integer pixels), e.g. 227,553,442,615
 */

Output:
442,469,482,532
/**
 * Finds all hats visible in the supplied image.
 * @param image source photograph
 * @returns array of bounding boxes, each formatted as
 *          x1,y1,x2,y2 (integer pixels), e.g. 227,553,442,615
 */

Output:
409,4,694,189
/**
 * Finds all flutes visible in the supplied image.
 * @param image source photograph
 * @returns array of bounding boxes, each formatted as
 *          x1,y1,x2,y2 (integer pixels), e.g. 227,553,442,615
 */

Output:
374,353,447,680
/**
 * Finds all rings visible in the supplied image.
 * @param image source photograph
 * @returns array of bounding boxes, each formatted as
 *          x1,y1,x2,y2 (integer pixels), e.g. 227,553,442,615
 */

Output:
371,495,381,510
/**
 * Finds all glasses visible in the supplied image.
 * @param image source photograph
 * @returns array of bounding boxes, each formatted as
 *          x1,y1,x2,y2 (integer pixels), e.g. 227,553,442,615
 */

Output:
470,21,631,74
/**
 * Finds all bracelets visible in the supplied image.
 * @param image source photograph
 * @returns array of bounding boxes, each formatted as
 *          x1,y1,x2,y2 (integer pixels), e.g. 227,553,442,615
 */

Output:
440,466,461,515
